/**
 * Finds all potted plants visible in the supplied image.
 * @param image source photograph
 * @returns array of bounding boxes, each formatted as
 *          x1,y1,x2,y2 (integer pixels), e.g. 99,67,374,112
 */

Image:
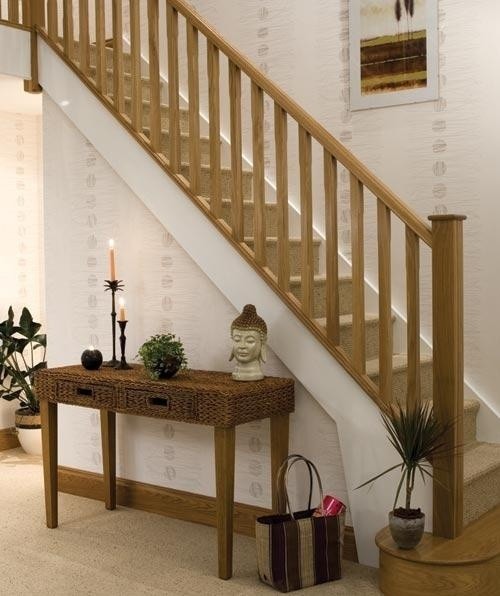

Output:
132,332,187,380
350,394,465,551
0,303,55,455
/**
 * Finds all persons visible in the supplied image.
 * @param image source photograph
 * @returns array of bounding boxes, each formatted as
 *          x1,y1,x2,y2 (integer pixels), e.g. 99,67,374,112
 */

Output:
228,304,267,381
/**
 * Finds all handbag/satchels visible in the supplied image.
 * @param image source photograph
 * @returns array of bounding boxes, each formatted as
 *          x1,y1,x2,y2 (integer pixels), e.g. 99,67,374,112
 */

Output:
253,453,347,592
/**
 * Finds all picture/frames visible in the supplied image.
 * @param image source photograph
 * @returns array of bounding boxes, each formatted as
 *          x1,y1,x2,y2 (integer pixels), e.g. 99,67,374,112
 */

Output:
346,0,441,118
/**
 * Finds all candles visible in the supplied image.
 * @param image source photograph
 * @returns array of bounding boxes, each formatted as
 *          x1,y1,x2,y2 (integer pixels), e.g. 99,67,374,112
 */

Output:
116,296,128,321
104,233,120,282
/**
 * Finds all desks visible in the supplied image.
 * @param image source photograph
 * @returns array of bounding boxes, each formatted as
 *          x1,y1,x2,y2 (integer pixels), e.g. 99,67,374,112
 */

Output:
26,354,297,585
